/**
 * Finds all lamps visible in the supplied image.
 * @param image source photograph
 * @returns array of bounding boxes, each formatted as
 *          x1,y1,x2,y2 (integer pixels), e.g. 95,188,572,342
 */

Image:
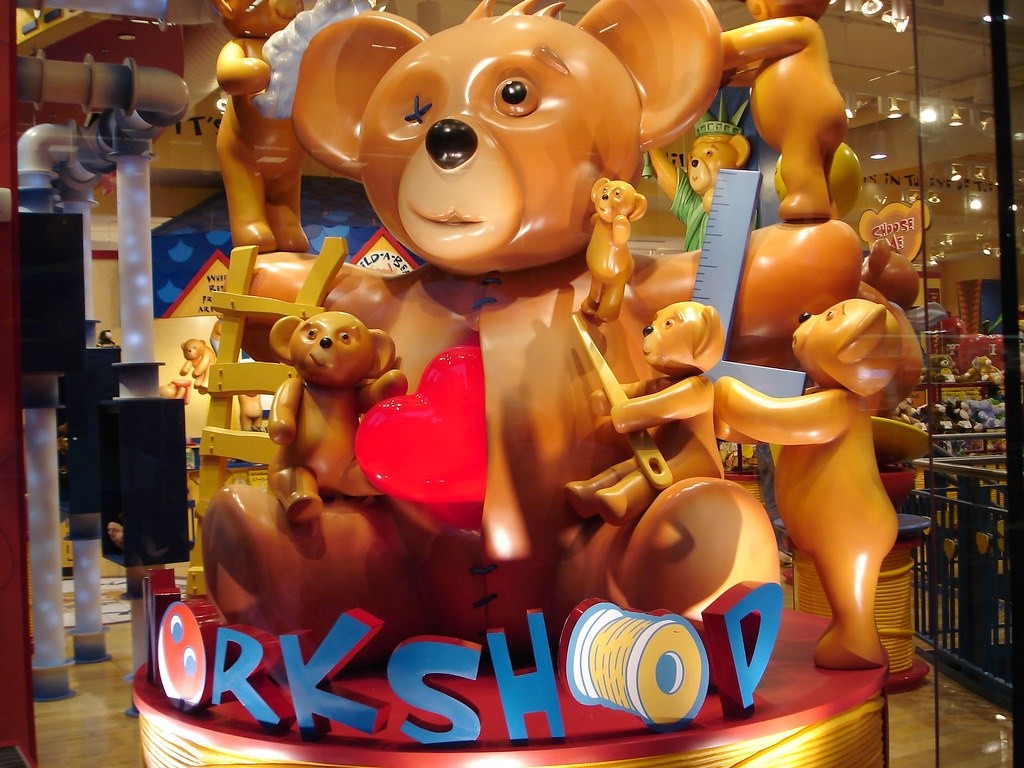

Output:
827,0,1024,271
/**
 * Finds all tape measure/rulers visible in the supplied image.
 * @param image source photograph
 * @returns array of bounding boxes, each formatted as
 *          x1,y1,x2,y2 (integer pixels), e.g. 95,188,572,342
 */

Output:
689,169,807,399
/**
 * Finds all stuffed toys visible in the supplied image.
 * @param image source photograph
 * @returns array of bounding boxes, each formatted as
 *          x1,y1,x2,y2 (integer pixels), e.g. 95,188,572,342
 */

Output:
893,350,1008,432
96,329,117,349
